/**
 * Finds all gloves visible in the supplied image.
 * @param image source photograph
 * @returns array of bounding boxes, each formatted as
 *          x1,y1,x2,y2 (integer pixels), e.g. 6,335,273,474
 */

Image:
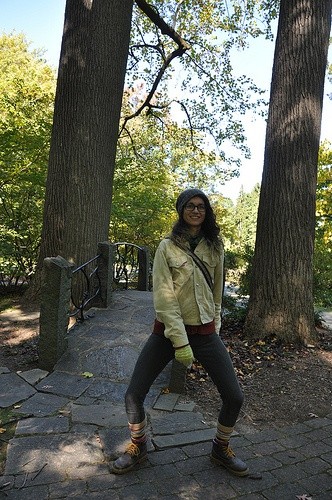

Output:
174,345,196,369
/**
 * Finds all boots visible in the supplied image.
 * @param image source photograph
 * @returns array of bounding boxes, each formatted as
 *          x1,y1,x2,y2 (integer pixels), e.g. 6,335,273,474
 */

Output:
108,442,147,475
208,441,250,477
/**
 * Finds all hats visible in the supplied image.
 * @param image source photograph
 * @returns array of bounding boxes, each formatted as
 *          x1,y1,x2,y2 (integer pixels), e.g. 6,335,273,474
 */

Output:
175,188,209,215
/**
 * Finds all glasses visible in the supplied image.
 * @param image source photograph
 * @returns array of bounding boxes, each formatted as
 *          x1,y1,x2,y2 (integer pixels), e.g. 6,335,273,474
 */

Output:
183,202,209,213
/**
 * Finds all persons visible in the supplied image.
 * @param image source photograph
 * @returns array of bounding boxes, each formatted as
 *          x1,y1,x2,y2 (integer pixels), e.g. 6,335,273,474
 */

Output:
110,189,250,476
